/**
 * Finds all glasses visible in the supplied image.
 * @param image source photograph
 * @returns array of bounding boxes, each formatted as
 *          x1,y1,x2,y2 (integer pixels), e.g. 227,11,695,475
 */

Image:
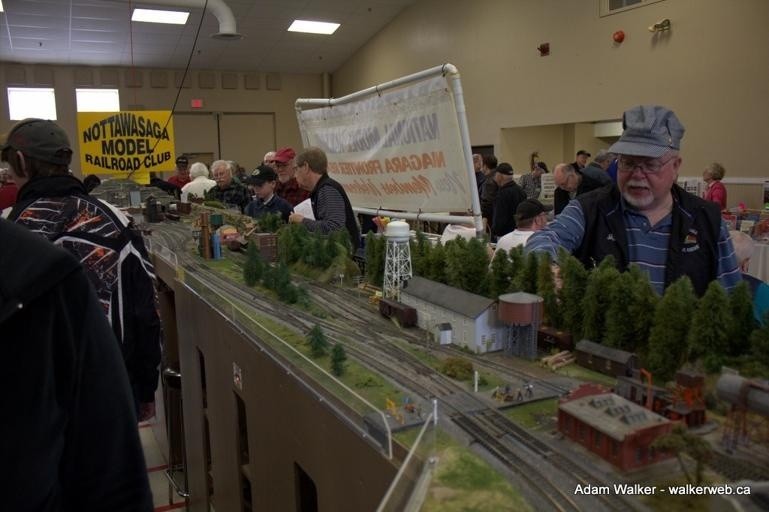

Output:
618,159,671,172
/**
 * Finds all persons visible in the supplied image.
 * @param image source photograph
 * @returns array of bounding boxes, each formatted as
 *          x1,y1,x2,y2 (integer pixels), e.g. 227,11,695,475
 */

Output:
179,161,218,203
491,162,529,236
527,104,743,311
273,147,310,206
167,157,191,188
227,160,249,184
287,146,359,255
437,212,488,247
573,144,618,181
553,163,606,270
244,165,293,224
0,216,155,512
472,153,486,184
697,161,727,211
517,162,549,198
69,173,101,194
264,151,278,170
1,168,19,215
478,154,499,211
0,118,162,421
207,160,253,215
489,198,553,272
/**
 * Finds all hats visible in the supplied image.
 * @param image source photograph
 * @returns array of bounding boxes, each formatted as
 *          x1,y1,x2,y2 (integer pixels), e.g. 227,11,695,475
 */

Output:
242,166,276,183
538,162,548,173
517,199,553,220
0,118,72,164
607,105,685,159
270,148,295,164
577,150,591,157
492,163,513,175
176,156,188,164
